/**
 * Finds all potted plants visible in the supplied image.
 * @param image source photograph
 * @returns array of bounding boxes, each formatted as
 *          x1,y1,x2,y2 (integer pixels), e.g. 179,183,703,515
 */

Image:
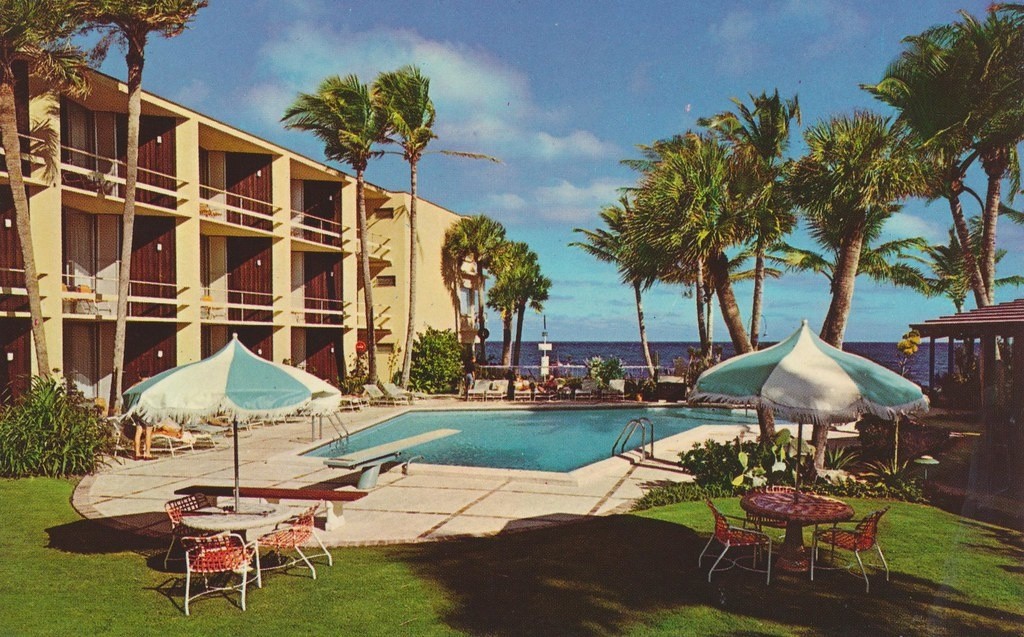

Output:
626,375,657,401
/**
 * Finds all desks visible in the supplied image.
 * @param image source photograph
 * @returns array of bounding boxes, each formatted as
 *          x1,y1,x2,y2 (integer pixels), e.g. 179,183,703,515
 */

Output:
739,491,855,579
179,501,293,548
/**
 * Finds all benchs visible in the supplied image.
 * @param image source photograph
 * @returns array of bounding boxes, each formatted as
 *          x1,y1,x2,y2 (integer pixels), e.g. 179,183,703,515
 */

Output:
174,484,369,533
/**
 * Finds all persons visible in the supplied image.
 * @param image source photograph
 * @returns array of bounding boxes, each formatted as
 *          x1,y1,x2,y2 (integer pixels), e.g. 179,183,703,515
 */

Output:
135,369,160,461
538,375,558,394
465,356,477,389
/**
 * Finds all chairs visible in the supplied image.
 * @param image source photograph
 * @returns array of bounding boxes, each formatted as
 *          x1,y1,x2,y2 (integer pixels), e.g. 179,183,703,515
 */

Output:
467,378,627,405
180,533,262,615
200,295,227,322
810,505,891,595
105,381,415,460
742,484,820,561
254,498,333,581
78,283,113,317
697,496,772,586
62,283,87,315
163,493,212,571
62,171,99,191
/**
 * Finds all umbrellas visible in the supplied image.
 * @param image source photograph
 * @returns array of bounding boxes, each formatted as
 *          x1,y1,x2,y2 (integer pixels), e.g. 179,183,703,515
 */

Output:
691,317,931,504
125,332,340,512
